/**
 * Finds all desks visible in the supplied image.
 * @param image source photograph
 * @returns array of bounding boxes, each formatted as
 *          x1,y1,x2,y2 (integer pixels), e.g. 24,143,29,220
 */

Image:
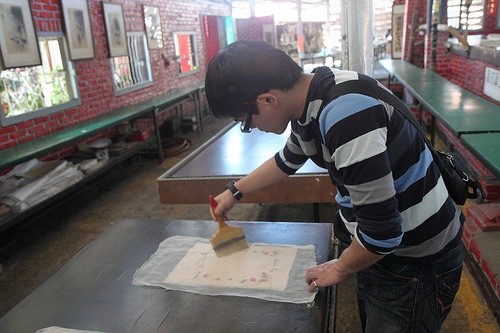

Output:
0,58,500,333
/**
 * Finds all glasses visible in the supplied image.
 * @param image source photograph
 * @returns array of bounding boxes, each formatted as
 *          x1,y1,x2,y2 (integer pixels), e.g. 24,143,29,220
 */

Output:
240,88,270,133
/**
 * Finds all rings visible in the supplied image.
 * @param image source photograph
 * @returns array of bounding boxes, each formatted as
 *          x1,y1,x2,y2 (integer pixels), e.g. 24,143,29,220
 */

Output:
314,281,317,287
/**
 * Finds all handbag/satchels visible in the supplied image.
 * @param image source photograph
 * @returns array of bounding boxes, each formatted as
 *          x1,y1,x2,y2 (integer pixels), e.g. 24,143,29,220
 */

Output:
434,150,469,206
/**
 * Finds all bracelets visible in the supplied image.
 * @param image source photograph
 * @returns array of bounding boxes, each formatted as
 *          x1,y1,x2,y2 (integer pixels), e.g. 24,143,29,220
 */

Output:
225,182,243,200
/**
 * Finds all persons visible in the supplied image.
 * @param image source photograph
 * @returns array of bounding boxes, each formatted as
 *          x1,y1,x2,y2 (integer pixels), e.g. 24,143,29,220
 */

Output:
204,40,466,333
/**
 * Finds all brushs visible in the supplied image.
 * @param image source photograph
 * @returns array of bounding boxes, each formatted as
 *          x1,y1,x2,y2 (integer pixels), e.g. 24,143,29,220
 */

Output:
207,194,251,257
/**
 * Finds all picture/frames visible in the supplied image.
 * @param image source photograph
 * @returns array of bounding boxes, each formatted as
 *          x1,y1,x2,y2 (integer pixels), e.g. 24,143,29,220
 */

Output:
58,0,96,62
101,1,130,58
0,0,43,71
142,4,165,51
